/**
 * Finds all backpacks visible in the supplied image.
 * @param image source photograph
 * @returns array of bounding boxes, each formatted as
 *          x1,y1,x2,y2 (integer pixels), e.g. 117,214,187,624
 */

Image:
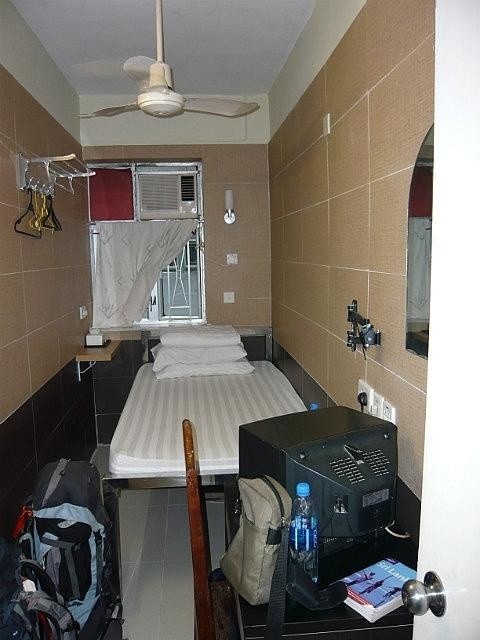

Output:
0,456,120,640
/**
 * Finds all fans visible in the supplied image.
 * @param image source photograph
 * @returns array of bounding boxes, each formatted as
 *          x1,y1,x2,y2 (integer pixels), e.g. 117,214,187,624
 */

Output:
73,0,265,129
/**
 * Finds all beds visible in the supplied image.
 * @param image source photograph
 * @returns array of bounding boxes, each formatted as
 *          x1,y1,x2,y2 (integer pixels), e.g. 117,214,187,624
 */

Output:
107,324,309,494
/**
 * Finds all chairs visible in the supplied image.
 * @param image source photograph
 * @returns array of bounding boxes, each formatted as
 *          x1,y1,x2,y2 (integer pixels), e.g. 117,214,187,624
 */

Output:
178,417,241,640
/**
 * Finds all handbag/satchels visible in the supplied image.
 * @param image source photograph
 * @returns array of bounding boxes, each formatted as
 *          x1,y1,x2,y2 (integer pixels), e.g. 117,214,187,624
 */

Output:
220,474,293,639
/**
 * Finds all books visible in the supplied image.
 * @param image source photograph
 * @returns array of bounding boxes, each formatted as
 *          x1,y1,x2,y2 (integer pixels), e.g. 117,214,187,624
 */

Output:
327,556,416,624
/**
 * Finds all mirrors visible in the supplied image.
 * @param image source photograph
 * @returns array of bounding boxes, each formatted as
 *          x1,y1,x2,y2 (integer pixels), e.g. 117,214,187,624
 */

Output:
404,125,429,361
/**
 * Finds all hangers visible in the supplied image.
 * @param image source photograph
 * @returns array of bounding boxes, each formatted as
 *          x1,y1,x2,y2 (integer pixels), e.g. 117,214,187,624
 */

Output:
14,186,65,242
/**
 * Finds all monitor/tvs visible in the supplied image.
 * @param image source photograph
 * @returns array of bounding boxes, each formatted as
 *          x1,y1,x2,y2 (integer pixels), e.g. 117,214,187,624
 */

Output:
239,407,407,558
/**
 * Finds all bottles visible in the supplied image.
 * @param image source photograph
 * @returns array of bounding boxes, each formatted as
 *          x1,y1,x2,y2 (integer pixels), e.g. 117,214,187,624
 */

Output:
288,482,319,601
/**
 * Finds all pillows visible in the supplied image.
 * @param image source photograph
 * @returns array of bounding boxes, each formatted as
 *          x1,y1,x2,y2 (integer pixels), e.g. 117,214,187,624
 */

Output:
152,322,256,381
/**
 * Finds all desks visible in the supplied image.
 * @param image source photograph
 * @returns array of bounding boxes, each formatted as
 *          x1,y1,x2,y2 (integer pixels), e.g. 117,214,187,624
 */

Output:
221,479,418,639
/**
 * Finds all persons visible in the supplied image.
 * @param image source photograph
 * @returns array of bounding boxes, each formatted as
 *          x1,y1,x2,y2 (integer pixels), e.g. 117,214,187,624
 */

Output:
346,571,375,589
358,579,384,596
383,585,402,598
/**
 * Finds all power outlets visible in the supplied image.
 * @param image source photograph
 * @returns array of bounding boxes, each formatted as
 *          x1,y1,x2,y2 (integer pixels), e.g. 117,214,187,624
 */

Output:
356,379,395,426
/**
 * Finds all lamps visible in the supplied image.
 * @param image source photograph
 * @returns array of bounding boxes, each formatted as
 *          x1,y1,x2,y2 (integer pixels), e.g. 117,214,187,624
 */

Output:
223,189,238,224
